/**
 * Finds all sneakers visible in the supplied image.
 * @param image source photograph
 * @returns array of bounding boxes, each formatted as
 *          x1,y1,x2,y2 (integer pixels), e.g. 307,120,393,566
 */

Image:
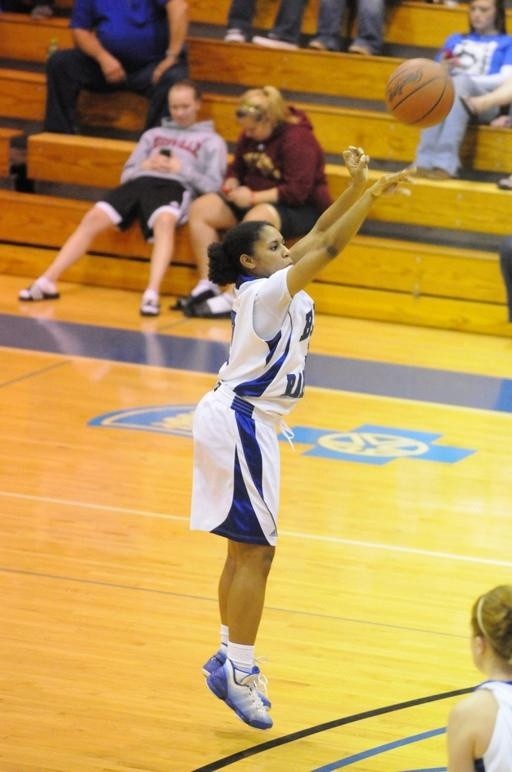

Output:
498,175,512,190
252,31,300,51
223,28,246,44
458,93,480,121
307,40,329,51
169,288,234,320
200,641,274,730
17,282,60,302
409,163,453,181
139,292,160,316
348,44,372,56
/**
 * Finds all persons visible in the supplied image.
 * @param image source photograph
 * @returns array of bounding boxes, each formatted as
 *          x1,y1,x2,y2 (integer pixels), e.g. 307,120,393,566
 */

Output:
461,73,512,130
441,582,512,771
406,1,512,180
166,83,337,320
183,141,422,730
309,0,399,56
216,0,308,49
39,1,185,136
8,76,229,321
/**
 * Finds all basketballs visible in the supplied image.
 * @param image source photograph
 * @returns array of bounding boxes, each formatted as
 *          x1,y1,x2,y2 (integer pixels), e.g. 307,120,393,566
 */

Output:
386,58,456,127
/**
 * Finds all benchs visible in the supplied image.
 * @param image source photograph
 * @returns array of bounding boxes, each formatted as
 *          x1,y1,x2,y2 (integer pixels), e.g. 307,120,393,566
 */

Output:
2,2,510,341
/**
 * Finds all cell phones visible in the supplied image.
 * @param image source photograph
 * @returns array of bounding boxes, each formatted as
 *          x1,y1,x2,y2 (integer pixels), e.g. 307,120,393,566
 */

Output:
160,148,170,157
